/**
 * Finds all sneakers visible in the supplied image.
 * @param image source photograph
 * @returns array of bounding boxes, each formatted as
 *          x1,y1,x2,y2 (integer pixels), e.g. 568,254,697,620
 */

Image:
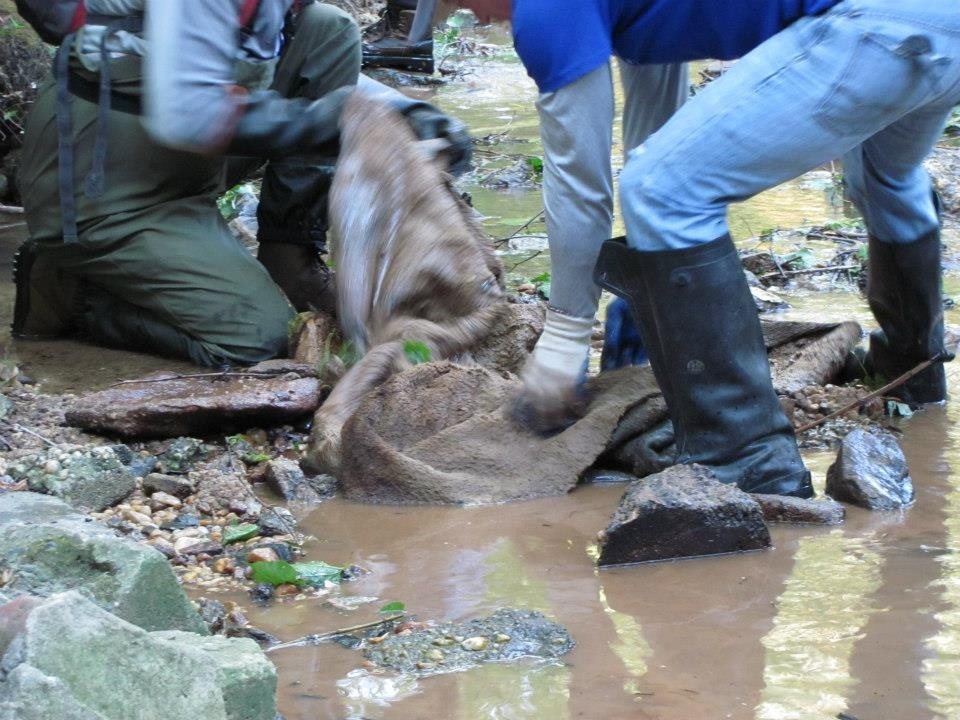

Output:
257,240,337,315
10,238,79,337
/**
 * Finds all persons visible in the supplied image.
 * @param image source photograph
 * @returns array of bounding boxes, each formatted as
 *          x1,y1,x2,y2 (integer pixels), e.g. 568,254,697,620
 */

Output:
1,1,471,372
393,0,960,497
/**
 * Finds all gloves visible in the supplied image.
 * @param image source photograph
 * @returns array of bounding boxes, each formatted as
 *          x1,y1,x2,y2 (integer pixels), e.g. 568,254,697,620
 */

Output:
392,98,474,179
508,310,595,438
599,295,648,372
231,85,349,156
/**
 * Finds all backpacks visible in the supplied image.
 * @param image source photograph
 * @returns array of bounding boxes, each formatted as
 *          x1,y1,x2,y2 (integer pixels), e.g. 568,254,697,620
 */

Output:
11,0,304,47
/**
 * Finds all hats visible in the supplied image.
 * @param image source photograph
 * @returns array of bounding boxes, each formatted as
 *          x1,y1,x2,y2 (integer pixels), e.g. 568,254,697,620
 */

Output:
405,0,453,45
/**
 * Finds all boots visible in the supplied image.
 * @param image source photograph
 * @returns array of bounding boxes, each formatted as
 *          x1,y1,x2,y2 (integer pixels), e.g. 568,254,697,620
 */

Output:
604,235,815,493
841,228,949,405
